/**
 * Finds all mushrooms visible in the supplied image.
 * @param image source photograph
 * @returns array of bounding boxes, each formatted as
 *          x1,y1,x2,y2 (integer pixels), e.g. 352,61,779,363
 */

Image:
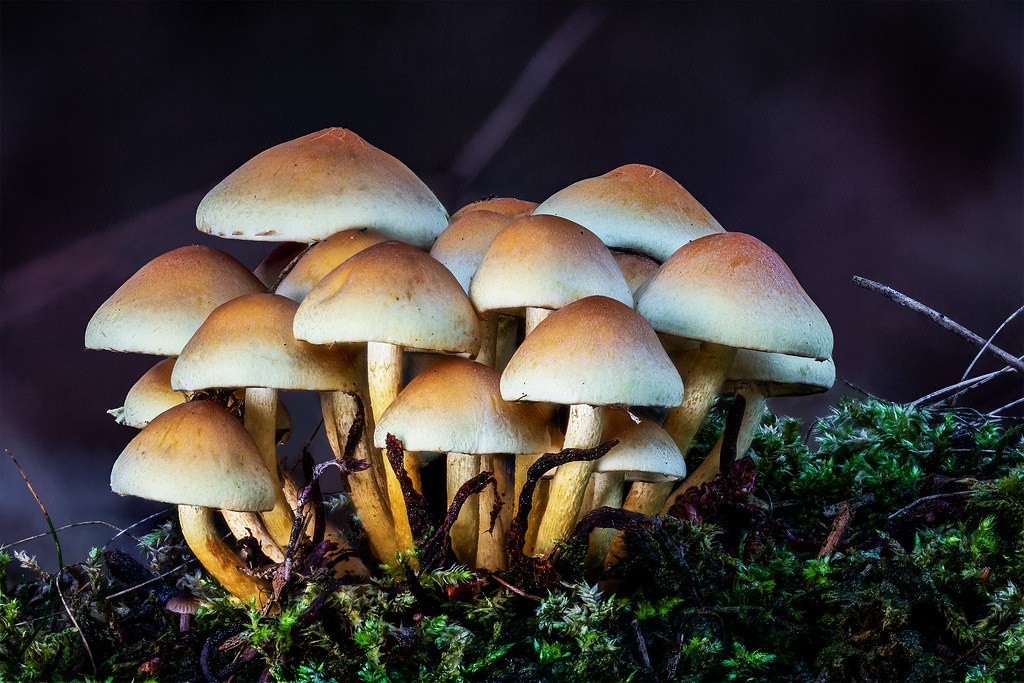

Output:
83,128,836,610
